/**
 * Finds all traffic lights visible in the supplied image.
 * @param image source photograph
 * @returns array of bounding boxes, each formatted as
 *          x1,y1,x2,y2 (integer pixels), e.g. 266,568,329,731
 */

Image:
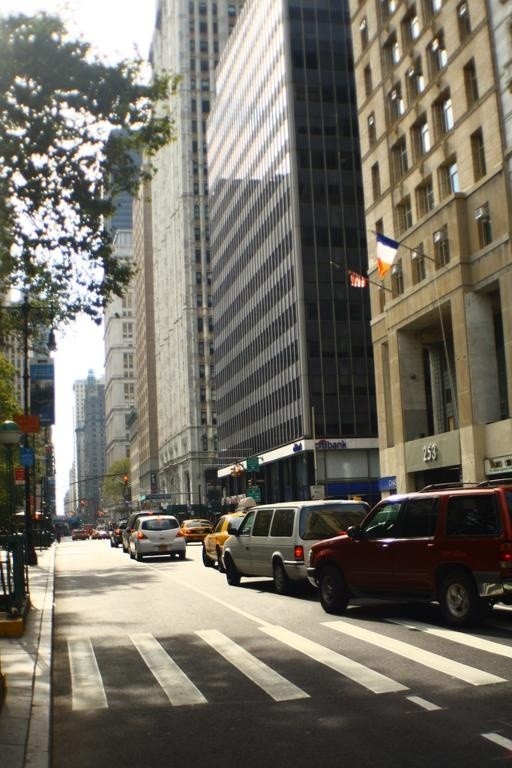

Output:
124,474,127,484
230,466,235,478
237,464,241,475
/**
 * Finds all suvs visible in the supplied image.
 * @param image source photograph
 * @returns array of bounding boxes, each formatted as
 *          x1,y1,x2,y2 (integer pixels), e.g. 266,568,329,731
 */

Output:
308,476,511,619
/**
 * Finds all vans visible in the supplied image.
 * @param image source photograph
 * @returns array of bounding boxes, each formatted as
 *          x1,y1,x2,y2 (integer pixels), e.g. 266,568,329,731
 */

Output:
220,498,370,591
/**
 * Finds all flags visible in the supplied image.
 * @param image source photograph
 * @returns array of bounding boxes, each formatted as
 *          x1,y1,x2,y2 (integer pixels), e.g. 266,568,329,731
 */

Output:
347,270,368,288
374,232,399,278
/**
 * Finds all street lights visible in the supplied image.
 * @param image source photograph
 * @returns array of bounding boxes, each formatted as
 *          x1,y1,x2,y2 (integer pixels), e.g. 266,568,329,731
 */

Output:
1,295,57,568
1,420,21,536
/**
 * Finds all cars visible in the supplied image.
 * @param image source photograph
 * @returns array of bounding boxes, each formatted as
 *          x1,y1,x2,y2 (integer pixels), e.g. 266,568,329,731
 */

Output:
0,509,244,568
